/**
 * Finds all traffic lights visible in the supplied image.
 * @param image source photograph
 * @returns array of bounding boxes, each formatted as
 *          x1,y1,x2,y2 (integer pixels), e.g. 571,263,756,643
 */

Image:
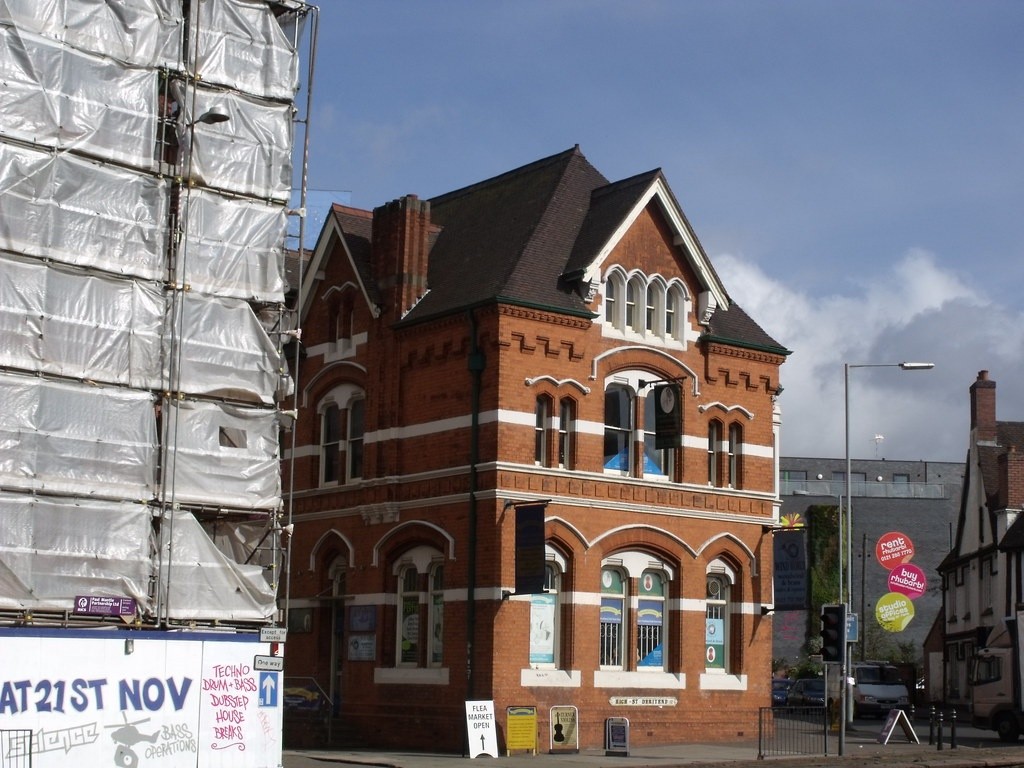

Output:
819,602,849,665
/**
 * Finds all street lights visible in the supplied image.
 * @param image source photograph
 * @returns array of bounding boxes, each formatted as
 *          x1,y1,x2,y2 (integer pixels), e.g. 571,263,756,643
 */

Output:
845,360,935,726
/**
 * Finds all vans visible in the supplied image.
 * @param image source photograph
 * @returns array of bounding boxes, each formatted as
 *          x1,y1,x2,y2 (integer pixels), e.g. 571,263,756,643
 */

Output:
824,660,910,724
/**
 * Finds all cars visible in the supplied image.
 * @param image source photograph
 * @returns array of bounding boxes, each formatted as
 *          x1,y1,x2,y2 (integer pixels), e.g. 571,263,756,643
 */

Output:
785,678,825,716
771,679,797,715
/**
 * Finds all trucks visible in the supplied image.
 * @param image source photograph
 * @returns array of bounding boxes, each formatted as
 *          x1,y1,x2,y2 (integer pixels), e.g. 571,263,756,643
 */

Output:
966,610,1024,744
